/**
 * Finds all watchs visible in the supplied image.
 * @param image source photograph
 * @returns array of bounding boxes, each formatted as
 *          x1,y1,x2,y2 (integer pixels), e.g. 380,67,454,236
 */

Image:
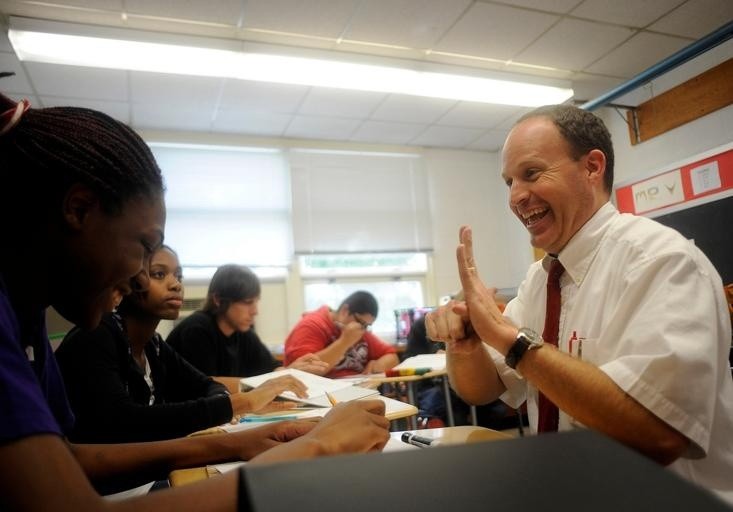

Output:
505,328,545,370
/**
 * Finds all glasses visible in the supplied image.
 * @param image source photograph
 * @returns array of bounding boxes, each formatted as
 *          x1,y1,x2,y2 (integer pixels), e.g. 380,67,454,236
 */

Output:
350,309,373,330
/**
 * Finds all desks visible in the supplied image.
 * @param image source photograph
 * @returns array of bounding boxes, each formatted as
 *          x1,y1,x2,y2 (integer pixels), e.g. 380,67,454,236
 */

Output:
213,353,480,427
168,424,512,487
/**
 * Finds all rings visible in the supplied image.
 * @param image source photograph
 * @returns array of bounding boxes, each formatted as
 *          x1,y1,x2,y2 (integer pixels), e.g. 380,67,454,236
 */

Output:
466,267,476,273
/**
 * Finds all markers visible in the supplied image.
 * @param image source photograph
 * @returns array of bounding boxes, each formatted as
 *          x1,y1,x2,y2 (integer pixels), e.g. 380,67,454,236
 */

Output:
401,432,438,447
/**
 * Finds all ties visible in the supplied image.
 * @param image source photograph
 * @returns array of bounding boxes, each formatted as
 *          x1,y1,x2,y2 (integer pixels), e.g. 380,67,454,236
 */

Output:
537,260,565,437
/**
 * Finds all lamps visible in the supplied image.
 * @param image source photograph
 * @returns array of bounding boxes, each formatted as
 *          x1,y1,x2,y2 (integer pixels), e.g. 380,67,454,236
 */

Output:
6,15,576,108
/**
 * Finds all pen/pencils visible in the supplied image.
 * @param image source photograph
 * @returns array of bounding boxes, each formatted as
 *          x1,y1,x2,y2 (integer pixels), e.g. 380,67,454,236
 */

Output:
239,414,298,424
578,337,587,361
569,331,577,353
325,390,337,407
334,320,346,330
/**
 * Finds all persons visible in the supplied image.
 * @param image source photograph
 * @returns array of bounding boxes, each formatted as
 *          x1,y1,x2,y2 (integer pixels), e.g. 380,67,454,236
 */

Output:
425,105,732,505
0,92,393,512
54,245,309,443
165,264,331,395
402,314,446,419
284,290,401,377
